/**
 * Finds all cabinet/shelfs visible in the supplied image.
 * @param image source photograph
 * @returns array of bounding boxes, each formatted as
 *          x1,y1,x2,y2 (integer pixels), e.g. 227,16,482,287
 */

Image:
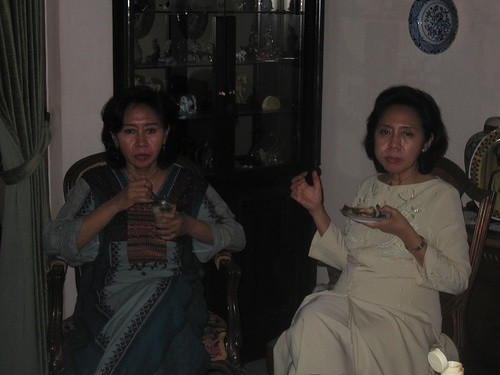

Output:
113,0,324,363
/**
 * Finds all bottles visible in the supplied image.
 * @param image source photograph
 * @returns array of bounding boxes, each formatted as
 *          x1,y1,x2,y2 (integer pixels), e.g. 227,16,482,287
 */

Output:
428,349,464,375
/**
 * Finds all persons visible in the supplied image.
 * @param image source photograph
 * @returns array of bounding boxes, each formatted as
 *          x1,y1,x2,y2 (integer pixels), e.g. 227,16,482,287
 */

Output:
273,85,472,375
43,84,246,375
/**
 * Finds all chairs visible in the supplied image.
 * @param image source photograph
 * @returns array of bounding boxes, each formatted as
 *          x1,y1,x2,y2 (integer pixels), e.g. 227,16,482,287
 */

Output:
46,151,241,375
440,170,496,375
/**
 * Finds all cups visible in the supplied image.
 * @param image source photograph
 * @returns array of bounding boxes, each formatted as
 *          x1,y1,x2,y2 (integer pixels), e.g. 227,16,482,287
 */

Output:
152,195,177,230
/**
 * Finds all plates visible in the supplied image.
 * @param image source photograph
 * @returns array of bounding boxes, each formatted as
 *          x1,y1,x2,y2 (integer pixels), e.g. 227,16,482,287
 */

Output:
408,0,458,54
339,207,379,225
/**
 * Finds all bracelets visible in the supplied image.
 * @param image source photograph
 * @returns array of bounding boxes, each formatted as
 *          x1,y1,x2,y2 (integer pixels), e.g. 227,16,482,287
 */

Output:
406,235,426,251
